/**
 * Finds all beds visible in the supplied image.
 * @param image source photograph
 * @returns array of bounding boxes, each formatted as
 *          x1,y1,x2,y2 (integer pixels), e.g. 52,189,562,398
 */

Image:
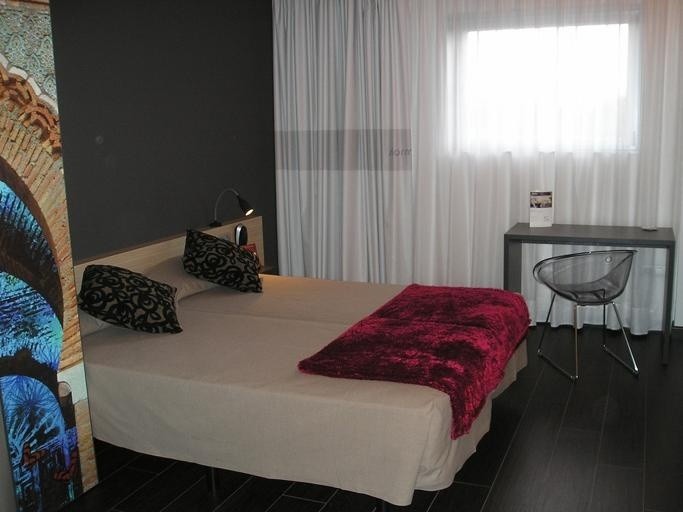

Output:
73,215,532,511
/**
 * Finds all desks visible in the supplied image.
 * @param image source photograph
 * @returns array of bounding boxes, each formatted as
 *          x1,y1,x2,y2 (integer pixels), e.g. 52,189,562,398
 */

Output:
503,223,676,364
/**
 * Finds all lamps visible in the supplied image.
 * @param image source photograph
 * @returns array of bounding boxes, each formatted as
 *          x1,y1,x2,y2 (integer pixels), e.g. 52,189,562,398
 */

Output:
208,187,255,227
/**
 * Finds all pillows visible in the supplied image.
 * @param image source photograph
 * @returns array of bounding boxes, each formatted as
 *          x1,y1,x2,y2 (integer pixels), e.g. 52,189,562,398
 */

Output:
182,227,262,294
75,264,182,335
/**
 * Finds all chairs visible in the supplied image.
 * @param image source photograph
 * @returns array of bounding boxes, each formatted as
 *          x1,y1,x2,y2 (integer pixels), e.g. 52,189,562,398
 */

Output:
532,250,640,384
